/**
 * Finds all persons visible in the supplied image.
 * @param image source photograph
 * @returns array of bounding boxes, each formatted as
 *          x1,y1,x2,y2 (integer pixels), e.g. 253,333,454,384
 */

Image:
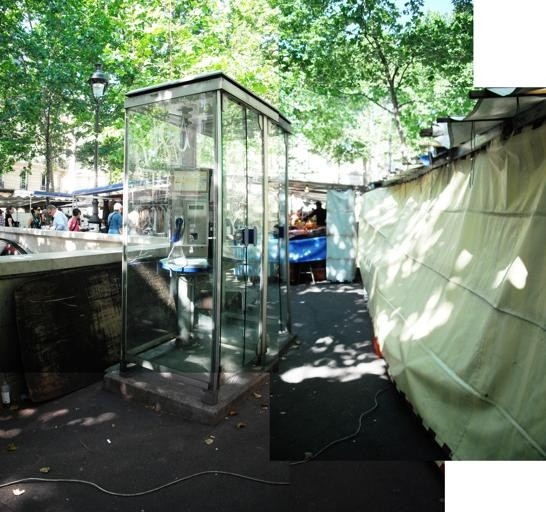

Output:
288,200,326,239
0,200,168,237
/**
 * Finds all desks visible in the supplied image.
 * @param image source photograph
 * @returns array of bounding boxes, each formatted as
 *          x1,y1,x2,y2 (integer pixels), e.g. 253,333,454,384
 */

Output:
289,234,330,287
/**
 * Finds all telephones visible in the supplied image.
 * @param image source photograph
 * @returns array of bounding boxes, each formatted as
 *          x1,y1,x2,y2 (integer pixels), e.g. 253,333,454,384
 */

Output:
172,199,208,247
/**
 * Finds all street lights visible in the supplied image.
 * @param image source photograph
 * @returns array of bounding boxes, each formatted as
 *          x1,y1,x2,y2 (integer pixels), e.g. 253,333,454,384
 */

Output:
86,64,108,232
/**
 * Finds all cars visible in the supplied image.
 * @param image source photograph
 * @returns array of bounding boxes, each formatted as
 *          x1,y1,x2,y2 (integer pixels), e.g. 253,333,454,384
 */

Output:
0,238,33,255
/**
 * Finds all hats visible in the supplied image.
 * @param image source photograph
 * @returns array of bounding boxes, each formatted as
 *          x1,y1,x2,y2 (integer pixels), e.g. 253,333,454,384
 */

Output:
114,203,122,210
